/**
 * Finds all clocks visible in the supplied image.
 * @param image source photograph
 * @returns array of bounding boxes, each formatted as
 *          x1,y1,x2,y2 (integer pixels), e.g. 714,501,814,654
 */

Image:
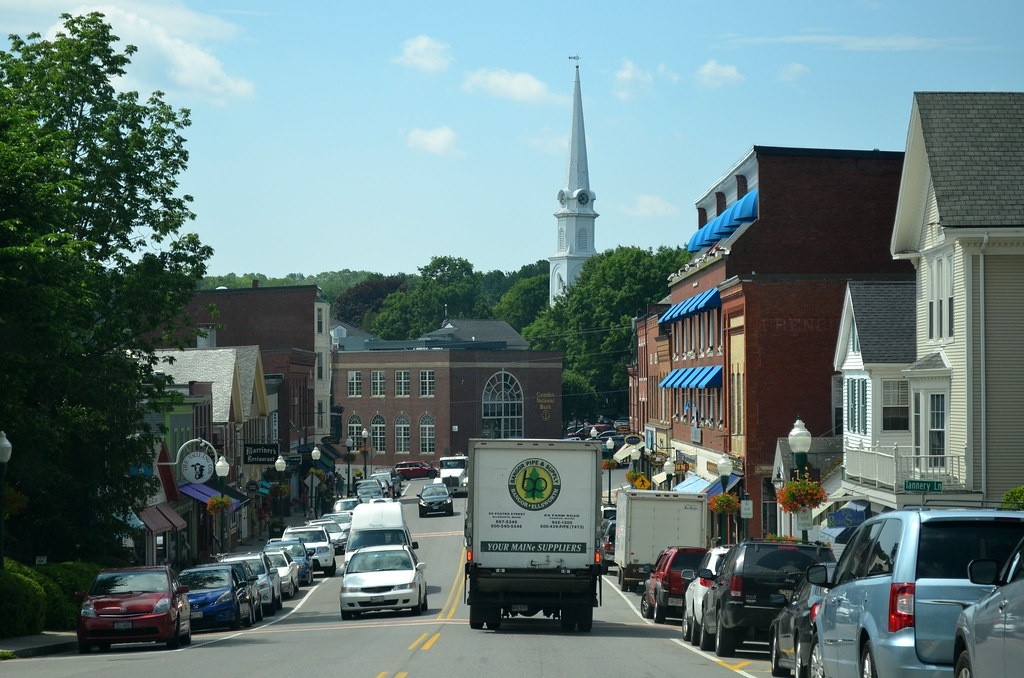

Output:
578,193,590,205
560,192,567,207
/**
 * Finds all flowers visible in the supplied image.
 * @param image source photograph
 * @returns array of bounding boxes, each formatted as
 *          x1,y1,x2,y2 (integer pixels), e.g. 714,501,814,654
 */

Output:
777,478,828,512
707,493,739,513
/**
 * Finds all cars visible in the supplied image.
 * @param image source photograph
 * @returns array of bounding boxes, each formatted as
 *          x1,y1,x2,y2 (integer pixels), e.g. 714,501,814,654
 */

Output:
74,566,192,653
177,561,263,631
768,562,837,678
248,551,299,597
335,544,427,620
566,425,625,448
953,537,1024,677
416,483,453,518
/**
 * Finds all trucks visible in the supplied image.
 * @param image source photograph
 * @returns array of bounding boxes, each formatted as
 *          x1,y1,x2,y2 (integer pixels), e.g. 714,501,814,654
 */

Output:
464,438,604,632
616,488,708,592
433,457,468,497
339,502,419,567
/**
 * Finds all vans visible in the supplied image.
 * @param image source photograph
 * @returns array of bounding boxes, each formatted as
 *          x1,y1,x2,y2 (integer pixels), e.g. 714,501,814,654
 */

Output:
807,510,1024,678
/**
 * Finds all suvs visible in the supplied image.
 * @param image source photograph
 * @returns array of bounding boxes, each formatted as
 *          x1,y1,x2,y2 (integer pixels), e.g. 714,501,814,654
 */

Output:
263,471,402,587
641,537,838,657
395,461,438,481
602,505,616,575
219,551,282,616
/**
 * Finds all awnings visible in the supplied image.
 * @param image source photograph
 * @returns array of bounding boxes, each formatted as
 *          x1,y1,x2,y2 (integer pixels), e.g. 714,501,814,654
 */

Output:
821,502,870,539
688,288,721,314
689,366,714,388
658,300,686,324
321,446,337,460
326,444,343,458
298,448,326,470
139,507,173,536
614,443,643,462
698,365,722,389
673,368,695,389
681,366,704,389
204,479,251,509
156,503,187,531
666,368,687,388
179,482,241,516
703,208,738,242
722,189,758,228
686,220,716,253
811,487,847,526
308,444,334,469
672,292,704,320
698,473,741,498
651,471,677,486
658,368,679,388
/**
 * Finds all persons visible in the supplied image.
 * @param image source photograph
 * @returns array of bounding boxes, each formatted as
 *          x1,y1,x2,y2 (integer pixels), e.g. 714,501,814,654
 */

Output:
301,469,347,521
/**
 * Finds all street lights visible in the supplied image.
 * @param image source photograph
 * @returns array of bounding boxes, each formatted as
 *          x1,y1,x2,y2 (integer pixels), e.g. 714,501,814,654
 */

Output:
275,455,290,518
717,451,735,546
346,435,353,498
788,414,811,545
0,430,12,568
606,438,614,504
362,428,369,479
215,455,229,553
311,446,321,517
